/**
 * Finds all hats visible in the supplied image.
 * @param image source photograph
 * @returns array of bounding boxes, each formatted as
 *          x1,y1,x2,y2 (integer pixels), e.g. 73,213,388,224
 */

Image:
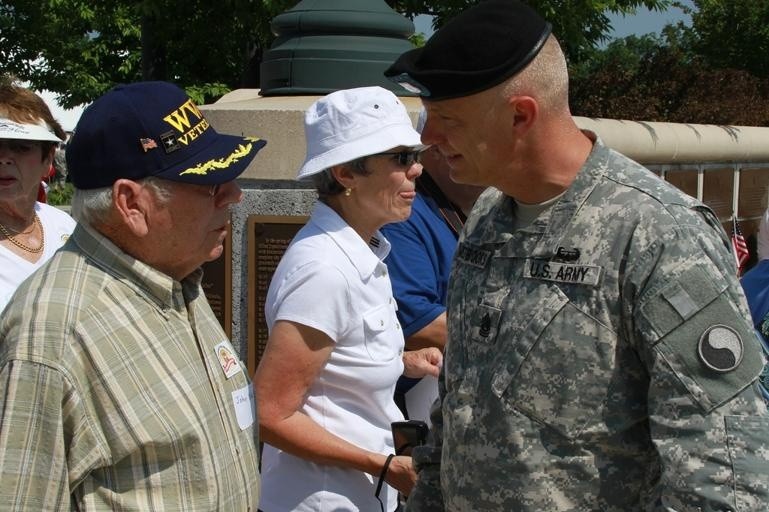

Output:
0,114,64,142
384,0,552,101
296,85,432,180
65,81,267,189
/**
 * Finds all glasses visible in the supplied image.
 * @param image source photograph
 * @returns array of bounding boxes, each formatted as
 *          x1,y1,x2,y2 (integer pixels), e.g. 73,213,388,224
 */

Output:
378,151,421,165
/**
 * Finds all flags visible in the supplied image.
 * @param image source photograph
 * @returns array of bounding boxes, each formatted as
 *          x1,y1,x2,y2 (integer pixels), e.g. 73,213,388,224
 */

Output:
726,212,751,275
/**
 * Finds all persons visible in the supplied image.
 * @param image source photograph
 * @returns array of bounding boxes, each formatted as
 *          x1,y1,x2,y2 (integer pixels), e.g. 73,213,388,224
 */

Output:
1,80,79,318
0,78,270,512
375,104,475,433
379,0,769,512
755,204,769,265
247,83,444,512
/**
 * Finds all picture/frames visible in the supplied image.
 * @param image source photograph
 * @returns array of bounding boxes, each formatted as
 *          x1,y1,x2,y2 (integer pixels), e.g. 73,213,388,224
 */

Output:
247,214,312,382
203,214,232,342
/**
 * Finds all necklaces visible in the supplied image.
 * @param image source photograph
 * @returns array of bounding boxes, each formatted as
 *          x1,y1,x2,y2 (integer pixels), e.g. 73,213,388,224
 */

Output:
0,214,36,235
0,209,46,255
